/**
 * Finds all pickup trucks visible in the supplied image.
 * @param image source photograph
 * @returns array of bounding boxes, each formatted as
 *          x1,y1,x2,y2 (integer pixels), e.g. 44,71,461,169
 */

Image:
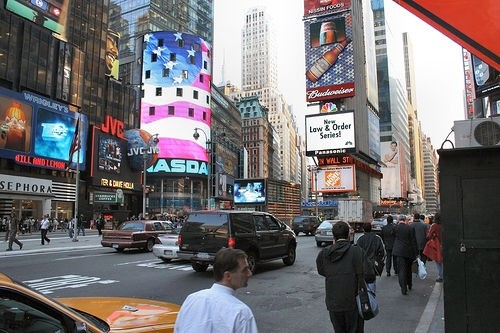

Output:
152,233,183,263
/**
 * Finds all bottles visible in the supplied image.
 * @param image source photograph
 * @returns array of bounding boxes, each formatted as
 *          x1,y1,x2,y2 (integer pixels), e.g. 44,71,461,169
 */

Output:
307,38,351,82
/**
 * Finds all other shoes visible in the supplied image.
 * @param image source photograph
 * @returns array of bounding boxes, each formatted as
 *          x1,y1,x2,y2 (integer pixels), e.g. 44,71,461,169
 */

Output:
20,244,24,249
436,278,443,282
6,249,12,251
387,273,390,276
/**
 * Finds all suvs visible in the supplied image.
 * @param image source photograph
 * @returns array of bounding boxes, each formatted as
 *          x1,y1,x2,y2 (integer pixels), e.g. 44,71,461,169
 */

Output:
291,216,321,236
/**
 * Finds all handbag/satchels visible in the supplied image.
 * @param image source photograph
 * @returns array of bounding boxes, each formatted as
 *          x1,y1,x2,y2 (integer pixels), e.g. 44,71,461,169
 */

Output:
411,259,419,273
368,256,385,281
417,257,427,280
355,277,378,319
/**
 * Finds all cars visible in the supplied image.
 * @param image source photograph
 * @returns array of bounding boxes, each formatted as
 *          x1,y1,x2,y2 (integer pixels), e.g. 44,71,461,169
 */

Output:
314,220,356,247
371,214,400,231
100,219,174,253
1,268,182,332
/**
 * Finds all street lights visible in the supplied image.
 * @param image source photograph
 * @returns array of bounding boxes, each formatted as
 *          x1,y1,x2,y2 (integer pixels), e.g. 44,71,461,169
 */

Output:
209,127,226,197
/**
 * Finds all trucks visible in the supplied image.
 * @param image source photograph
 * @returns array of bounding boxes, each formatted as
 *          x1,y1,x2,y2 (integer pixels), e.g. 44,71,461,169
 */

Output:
337,198,374,232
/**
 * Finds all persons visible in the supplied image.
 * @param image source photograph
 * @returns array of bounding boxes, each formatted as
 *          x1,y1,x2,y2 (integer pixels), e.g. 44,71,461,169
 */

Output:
316,220,376,333
61,215,85,238
383,141,398,164
381,215,398,276
40,215,50,245
411,212,444,283
0,214,58,242
105,29,120,80
97,217,105,235
5,217,23,251
391,216,418,295
357,222,386,298
174,248,258,333
242,183,264,202
118,213,188,227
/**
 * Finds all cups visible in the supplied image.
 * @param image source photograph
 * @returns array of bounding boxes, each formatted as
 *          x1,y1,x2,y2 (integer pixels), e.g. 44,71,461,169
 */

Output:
319,22,337,45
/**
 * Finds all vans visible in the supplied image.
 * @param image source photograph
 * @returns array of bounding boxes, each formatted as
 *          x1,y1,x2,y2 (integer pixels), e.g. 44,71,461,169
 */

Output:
176,209,298,273
